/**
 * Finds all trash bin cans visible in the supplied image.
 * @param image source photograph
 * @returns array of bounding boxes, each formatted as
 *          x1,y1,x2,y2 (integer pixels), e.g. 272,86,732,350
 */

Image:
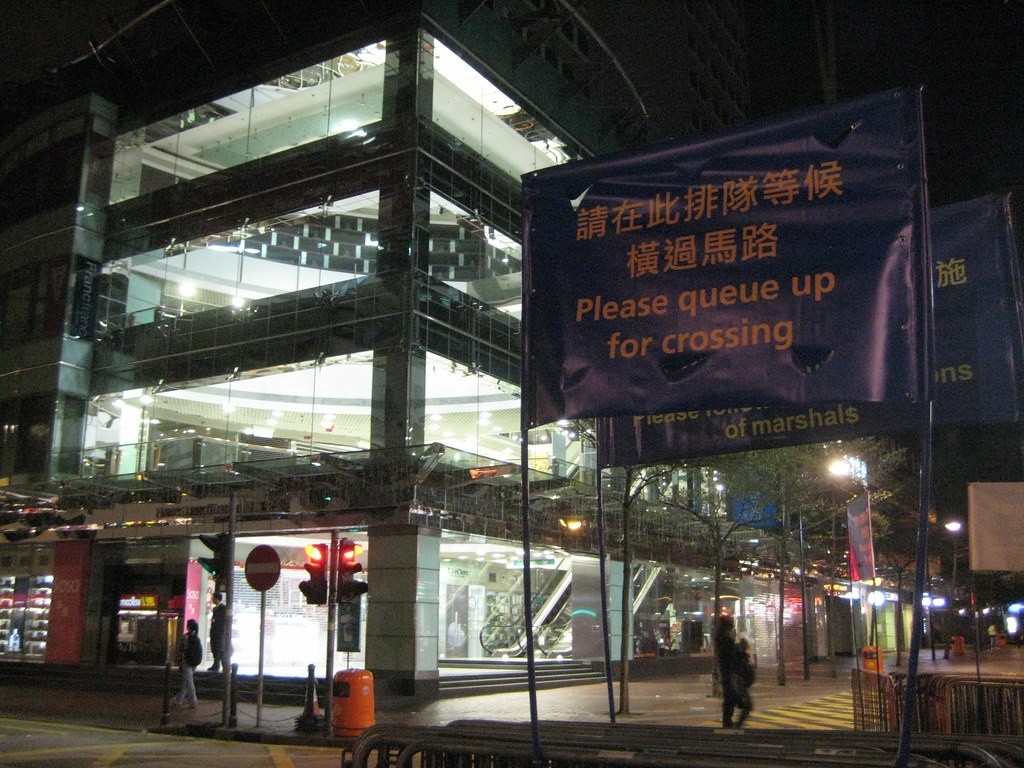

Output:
996,633,1008,650
332,667,375,739
861,643,884,674
950,636,967,657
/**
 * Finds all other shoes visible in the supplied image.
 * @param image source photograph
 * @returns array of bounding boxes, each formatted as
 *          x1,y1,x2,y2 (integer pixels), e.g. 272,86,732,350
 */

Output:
208,666,219,671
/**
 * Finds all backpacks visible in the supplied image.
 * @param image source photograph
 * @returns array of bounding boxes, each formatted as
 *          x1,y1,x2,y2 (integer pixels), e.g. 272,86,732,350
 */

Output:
183,633,202,666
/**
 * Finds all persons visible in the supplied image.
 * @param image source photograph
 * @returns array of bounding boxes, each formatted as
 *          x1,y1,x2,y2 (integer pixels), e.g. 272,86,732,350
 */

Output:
988,621,997,649
714,616,752,727
171,619,202,706
208,593,226,670
